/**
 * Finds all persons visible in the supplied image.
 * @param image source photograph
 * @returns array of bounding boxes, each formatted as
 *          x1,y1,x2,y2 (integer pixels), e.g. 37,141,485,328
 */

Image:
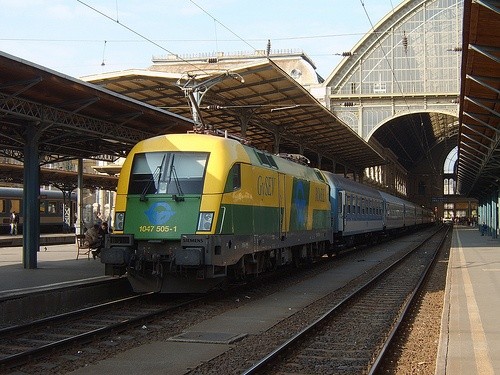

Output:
10,210,19,236
83,222,112,260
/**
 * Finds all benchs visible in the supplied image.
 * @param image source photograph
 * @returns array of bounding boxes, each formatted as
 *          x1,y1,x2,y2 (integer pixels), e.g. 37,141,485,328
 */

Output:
76,235,92,260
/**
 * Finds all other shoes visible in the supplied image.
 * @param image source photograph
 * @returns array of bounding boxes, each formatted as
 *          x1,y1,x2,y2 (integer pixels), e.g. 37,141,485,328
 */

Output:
92,251,97,260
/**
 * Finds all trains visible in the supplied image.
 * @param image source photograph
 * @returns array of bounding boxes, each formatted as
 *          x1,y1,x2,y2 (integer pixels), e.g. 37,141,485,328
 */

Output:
99,69,436,296
0,187,77,235
